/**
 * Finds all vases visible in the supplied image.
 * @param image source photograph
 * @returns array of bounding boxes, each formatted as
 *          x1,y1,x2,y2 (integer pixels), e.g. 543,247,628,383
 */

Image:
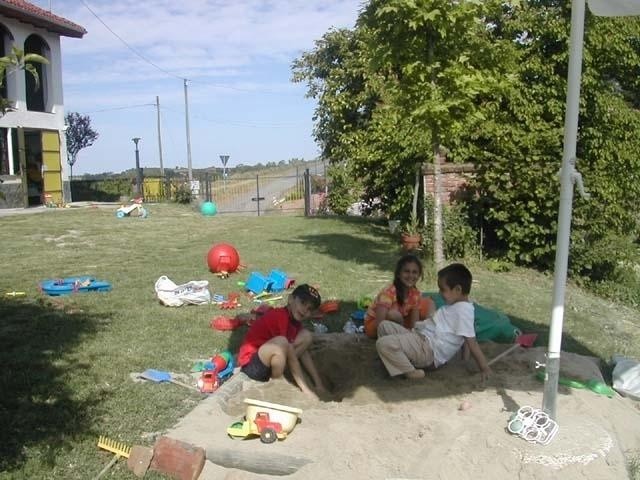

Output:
388,220,401,235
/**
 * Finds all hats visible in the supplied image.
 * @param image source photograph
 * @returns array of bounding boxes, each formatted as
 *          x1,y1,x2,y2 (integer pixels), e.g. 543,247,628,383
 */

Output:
293,284,321,310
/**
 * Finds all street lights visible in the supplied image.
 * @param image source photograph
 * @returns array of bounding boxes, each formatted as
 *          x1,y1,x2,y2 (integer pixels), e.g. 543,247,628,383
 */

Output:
132,137,141,201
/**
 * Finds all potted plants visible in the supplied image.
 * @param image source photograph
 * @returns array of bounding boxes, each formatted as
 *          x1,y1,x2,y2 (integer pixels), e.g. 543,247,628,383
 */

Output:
397,211,421,249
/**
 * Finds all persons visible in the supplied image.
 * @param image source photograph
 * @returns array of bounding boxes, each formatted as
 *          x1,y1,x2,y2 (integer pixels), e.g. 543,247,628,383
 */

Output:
375,263,492,383
238,284,328,402
362,255,436,337
